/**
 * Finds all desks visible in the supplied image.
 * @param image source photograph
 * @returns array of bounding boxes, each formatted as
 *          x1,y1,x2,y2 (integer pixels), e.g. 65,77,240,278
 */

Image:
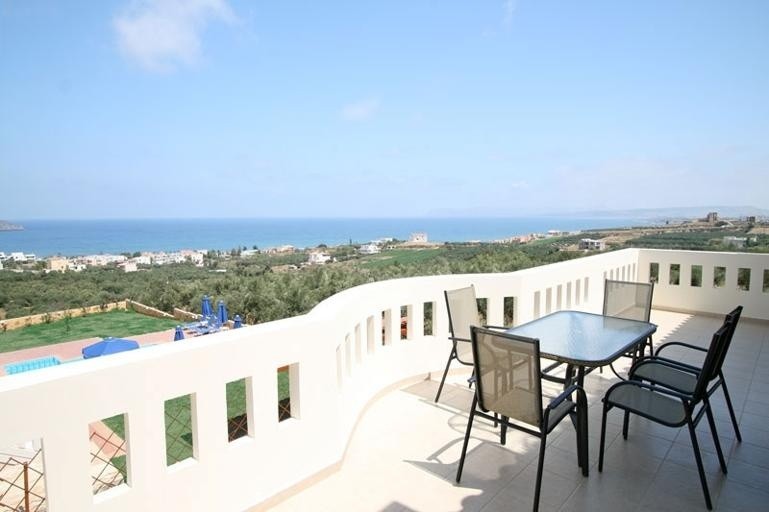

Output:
498,309,659,469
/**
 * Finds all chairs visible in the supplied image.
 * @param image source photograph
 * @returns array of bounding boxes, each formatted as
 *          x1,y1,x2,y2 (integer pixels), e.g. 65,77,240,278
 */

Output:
600,279,654,379
435,283,530,429
456,325,590,511
623,303,743,458
599,321,733,511
